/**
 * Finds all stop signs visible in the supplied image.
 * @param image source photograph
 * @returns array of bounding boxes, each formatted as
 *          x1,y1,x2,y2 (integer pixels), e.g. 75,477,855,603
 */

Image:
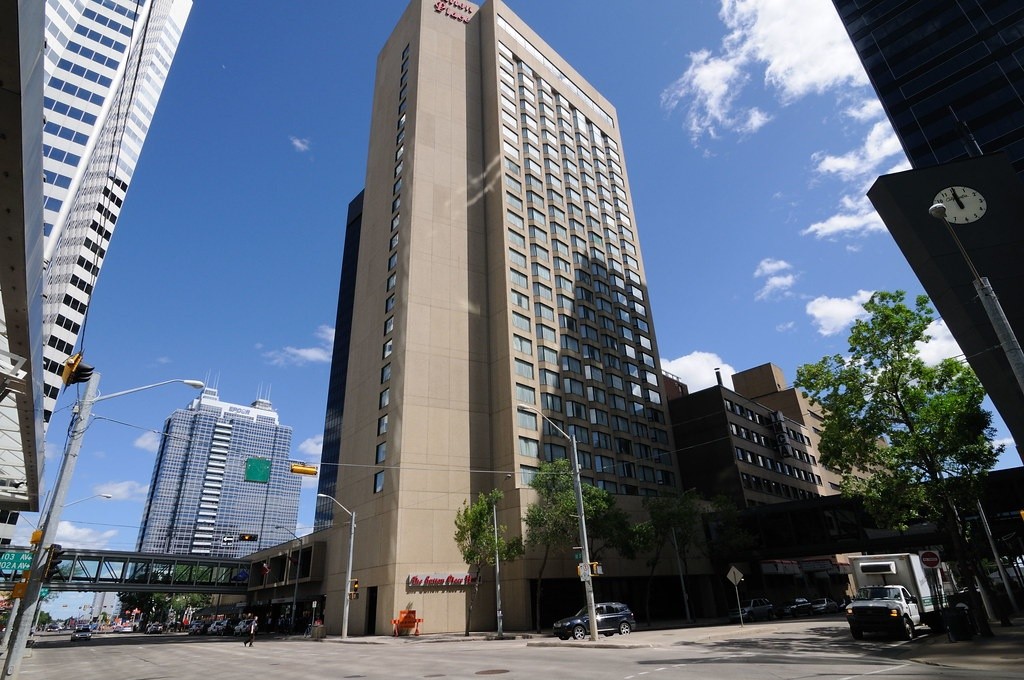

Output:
919,551,946,574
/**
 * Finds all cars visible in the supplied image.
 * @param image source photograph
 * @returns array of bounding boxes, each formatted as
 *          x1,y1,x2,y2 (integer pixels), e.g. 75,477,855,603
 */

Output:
189,618,243,636
70,626,93,642
145,622,165,635
46,624,59,632
775,598,815,620
112,625,133,633
812,597,840,616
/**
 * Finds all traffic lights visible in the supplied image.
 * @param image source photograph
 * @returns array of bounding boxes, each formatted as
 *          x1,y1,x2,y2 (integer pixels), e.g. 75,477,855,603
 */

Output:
592,562,604,576
352,592,360,599
41,544,64,584
61,352,95,395
352,581,359,592
239,534,259,543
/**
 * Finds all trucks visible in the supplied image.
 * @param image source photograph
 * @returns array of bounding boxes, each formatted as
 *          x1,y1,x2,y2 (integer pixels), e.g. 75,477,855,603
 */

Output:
847,553,978,643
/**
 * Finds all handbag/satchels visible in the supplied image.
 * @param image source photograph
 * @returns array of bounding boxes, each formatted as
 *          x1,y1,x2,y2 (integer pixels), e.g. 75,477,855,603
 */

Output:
246,622,252,633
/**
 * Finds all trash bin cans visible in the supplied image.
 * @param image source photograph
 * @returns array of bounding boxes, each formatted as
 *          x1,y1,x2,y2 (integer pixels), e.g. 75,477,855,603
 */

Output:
311,625,327,638
944,607,970,641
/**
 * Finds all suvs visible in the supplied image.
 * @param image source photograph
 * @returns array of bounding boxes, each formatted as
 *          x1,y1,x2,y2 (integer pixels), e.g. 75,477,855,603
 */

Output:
727,596,773,625
233,619,260,636
552,602,638,637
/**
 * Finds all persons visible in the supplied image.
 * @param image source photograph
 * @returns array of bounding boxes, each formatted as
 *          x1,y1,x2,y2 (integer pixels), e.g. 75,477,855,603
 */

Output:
244,616,258,647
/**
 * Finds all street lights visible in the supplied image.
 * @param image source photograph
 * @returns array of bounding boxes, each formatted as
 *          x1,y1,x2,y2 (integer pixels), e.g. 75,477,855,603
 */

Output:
273,525,302,635
0,369,204,680
1,490,112,655
316,492,358,641
492,474,512,637
670,486,695,626
517,403,602,639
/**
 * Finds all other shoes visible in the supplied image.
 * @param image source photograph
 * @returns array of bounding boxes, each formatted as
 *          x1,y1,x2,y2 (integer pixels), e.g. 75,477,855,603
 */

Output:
243,641,247,647
249,644,254,647
